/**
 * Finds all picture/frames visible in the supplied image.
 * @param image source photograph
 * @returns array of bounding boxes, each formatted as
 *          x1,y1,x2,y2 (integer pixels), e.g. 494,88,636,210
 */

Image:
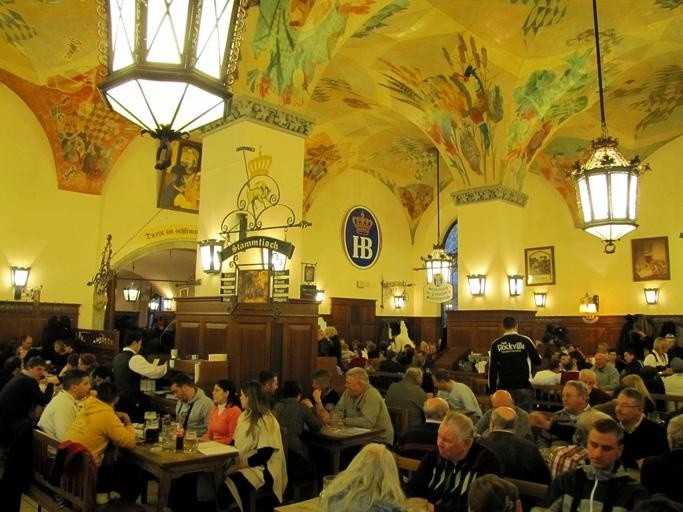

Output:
525,246,555,286
236,264,274,304
630,236,671,281
157,138,202,214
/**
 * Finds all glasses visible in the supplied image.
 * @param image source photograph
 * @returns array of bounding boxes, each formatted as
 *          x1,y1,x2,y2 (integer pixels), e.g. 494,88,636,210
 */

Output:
614,403,644,410
561,392,582,399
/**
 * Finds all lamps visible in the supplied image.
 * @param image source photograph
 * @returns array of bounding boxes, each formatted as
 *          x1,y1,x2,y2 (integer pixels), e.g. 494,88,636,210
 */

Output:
579,293,600,324
534,292,546,306
10,266,31,287
316,290,325,301
123,261,139,302
645,288,658,304
149,283,159,311
262,246,287,271
97,0,249,172
164,298,172,309
420,147,452,284
394,296,403,309
198,239,224,274
466,274,487,295
568,1,650,254
507,272,524,296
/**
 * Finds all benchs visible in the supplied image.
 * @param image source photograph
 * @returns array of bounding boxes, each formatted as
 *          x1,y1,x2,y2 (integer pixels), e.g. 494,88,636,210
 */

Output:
388,407,410,442
395,454,548,498
26,427,130,512
249,487,278,510
473,378,566,406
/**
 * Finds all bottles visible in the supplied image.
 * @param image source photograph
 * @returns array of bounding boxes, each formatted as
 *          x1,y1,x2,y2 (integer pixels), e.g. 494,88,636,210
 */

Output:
176,416,184,451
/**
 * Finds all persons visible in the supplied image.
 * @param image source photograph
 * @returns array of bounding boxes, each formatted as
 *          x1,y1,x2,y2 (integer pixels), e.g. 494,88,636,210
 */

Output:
609,350,622,371
530,418,653,512
591,386,625,411
271,382,322,504
383,368,432,436
547,343,592,369
644,338,672,375
586,353,619,390
472,389,535,445
257,370,278,408
301,370,338,414
624,350,644,373
148,317,166,336
619,315,653,350
401,414,497,512
642,415,682,512
637,366,664,408
61,382,143,512
313,368,392,451
469,476,523,511
168,374,215,436
550,411,621,477
113,333,168,422
557,359,579,387
207,381,288,512
607,388,669,471
598,343,609,358
577,369,610,403
334,333,442,372
550,380,610,444
665,334,682,368
618,375,654,414
532,358,560,386
0,357,56,463
4,333,39,372
39,370,92,484
316,443,407,511
430,370,483,428
399,398,452,460
659,320,677,337
195,381,242,508
487,318,543,412
476,406,551,483
49,337,117,397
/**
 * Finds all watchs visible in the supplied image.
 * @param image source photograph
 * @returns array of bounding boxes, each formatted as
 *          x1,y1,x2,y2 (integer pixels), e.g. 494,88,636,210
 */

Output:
314,399,320,404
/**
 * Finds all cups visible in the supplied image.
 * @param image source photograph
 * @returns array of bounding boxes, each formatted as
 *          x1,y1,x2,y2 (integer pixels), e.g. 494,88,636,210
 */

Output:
183,437,199,455
